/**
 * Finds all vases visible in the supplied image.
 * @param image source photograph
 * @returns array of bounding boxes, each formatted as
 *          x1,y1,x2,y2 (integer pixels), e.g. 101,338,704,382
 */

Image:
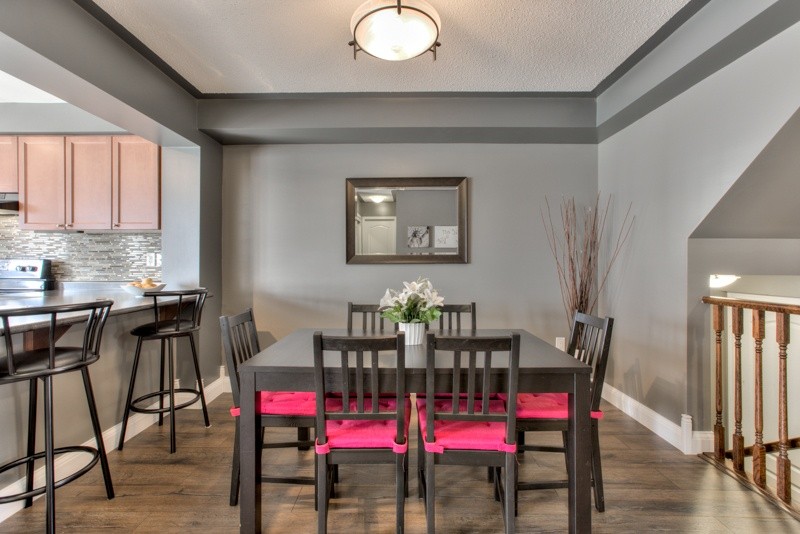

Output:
398,322,425,345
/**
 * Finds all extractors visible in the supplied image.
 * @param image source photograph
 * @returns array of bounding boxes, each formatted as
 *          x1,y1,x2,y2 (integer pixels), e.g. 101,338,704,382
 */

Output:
0,193,19,210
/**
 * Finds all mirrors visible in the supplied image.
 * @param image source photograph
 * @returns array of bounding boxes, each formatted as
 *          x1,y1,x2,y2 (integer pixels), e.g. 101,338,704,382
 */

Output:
345,177,468,264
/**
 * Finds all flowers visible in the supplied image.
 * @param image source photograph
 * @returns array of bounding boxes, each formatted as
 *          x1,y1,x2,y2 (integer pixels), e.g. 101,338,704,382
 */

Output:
378,275,443,324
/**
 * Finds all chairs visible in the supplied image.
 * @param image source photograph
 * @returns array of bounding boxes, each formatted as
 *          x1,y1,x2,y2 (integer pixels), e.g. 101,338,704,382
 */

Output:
332,301,409,397
494,309,614,516
417,333,520,534
417,302,496,397
219,307,318,511
314,331,412,534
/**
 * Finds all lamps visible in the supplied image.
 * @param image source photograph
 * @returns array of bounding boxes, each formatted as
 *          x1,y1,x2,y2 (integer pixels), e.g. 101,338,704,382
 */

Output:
348,0,442,61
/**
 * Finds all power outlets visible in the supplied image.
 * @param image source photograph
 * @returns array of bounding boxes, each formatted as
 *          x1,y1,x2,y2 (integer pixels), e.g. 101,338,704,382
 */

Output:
556,338,565,351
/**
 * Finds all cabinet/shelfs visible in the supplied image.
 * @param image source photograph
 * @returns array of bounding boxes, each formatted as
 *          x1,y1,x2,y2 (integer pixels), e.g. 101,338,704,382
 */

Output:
0,135,160,230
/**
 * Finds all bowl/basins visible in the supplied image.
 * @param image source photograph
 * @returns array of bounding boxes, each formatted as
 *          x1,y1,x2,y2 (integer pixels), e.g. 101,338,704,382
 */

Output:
121,284,166,298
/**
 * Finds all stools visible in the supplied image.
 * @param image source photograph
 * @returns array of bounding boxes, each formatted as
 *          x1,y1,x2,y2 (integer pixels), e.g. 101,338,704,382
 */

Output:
117,287,210,454
0,300,116,534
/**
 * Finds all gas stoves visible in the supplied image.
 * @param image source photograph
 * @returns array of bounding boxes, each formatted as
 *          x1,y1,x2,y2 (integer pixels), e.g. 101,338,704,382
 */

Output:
0,258,54,294
